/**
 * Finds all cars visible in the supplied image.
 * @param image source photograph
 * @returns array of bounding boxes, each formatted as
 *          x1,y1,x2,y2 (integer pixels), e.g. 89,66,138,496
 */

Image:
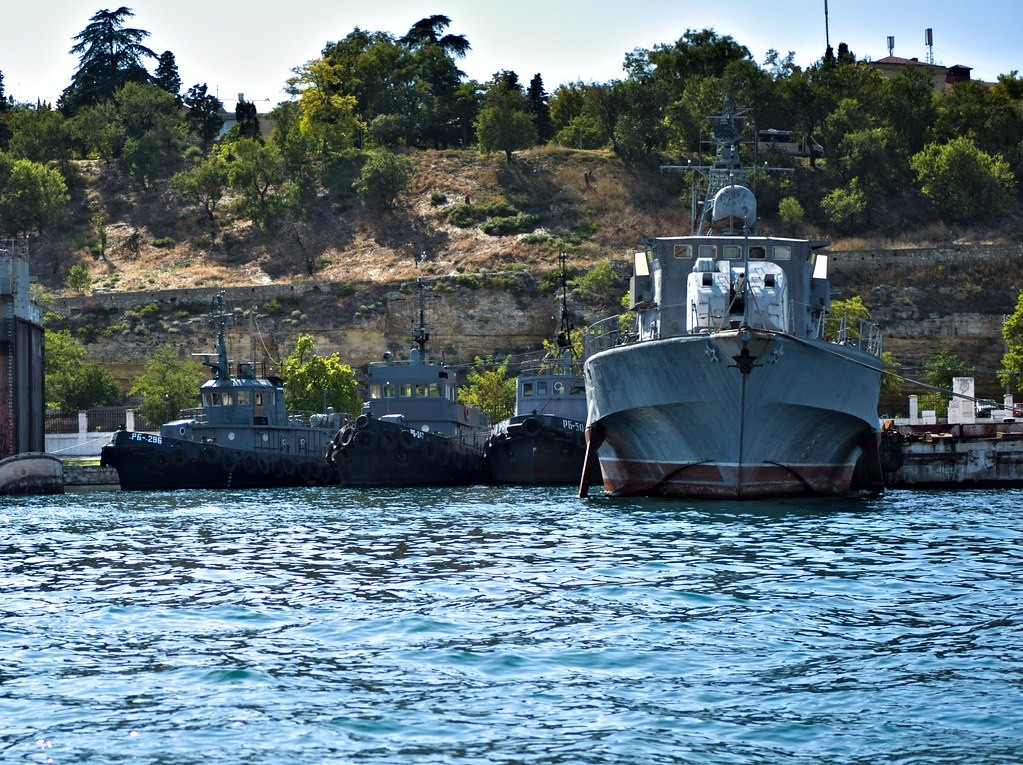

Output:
1013,403,1023,416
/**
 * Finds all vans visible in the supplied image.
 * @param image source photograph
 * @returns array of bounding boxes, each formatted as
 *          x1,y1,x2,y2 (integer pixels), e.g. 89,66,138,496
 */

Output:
757,129,824,157
974,399,998,418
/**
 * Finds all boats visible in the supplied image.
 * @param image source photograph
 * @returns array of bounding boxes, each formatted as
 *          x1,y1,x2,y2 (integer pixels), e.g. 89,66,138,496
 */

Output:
326,254,489,485
482,247,603,487
100,288,334,497
580,59,887,494
